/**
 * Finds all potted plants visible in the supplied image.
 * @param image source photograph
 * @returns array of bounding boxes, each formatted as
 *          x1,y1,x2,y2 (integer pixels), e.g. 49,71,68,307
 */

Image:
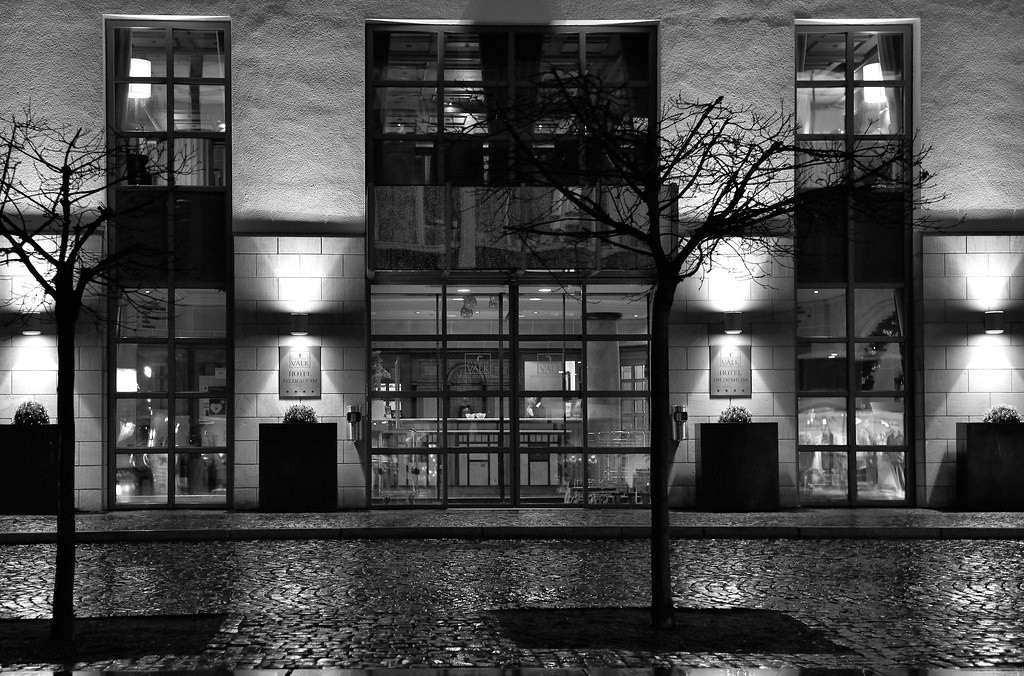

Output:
260,405,338,514
694,406,779,513
0,399,61,516
956,406,1024,512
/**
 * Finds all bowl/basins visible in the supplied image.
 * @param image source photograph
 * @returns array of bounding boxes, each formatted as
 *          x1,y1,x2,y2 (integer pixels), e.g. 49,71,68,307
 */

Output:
476,413,486,419
465,413,475,419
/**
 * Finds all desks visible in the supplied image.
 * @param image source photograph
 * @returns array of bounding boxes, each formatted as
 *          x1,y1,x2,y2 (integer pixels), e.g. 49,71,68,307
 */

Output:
572,486,617,503
